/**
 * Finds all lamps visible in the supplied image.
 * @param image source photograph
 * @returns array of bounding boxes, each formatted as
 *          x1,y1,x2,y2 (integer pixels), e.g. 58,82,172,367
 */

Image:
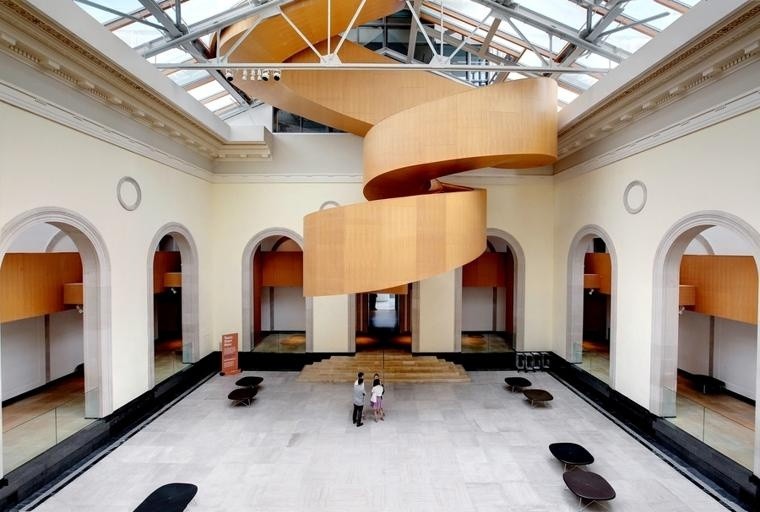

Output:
225,68,281,82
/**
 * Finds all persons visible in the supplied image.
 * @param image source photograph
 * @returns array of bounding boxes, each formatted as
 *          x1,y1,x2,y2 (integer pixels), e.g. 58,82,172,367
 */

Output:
371,373,385,416
371,378,384,422
352,377,366,427
353,372,367,418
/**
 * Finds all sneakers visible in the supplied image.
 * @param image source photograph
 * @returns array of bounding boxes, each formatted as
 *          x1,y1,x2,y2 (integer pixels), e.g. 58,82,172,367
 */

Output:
362,415,368,420
375,416,386,422
353,419,364,427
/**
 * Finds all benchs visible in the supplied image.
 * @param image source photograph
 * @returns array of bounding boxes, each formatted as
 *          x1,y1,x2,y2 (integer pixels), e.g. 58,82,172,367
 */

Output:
132,482,198,512
549,443,616,512
688,374,726,394
228,376,264,408
504,376,554,408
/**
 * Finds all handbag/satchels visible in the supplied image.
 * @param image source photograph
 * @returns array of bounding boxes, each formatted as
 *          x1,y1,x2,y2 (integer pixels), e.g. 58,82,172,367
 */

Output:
370,387,377,403
